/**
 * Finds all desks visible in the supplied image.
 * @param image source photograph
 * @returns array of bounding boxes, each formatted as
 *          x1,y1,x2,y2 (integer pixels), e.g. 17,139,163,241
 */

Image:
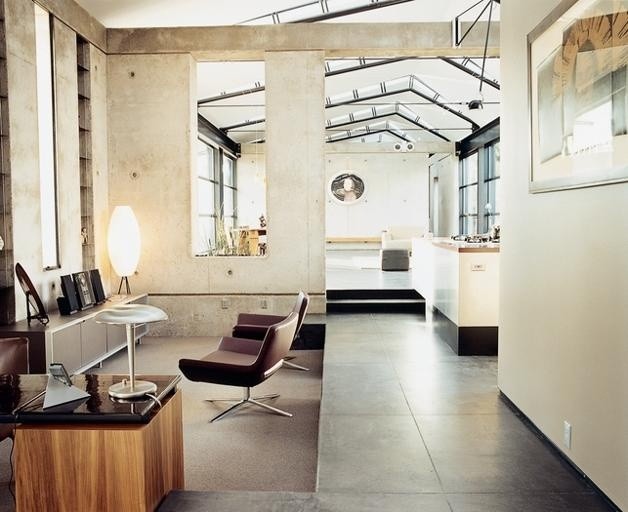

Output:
0,373,183,512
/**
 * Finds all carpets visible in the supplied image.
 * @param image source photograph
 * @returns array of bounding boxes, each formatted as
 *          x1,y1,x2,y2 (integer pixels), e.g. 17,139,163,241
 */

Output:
326,257,381,268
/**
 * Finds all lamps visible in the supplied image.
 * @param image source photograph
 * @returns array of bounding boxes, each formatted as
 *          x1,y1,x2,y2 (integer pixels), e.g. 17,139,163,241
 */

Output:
468,91,485,109
107,206,142,295
96,302,168,398
394,139,415,151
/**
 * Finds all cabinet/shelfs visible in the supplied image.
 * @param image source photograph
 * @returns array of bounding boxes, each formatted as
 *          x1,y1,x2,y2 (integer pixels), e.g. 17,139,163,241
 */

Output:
0,292,148,382
0,0,13,287
76,29,95,271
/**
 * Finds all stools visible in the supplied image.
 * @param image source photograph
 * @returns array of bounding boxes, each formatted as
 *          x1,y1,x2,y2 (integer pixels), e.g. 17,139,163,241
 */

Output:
383,250,409,271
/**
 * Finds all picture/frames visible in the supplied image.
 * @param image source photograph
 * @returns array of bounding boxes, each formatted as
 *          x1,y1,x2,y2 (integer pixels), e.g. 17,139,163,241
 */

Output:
527,0,628,195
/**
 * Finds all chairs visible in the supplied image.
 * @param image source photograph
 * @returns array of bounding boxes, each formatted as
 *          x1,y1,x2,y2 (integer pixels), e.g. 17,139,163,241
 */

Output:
178,292,312,424
0,337,29,376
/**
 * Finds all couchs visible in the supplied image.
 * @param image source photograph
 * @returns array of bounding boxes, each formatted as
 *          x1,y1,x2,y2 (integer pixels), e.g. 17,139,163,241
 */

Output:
381,224,432,249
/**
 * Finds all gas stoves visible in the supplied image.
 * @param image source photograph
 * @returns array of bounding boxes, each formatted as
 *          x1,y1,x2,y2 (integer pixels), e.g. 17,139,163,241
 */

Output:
448,233,500,245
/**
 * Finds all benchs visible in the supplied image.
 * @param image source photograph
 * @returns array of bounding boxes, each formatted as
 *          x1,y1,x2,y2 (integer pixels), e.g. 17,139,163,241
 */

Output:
325,236,382,256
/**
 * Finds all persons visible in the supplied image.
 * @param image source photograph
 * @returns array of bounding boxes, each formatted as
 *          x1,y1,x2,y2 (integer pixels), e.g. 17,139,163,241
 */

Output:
342,177,358,202
